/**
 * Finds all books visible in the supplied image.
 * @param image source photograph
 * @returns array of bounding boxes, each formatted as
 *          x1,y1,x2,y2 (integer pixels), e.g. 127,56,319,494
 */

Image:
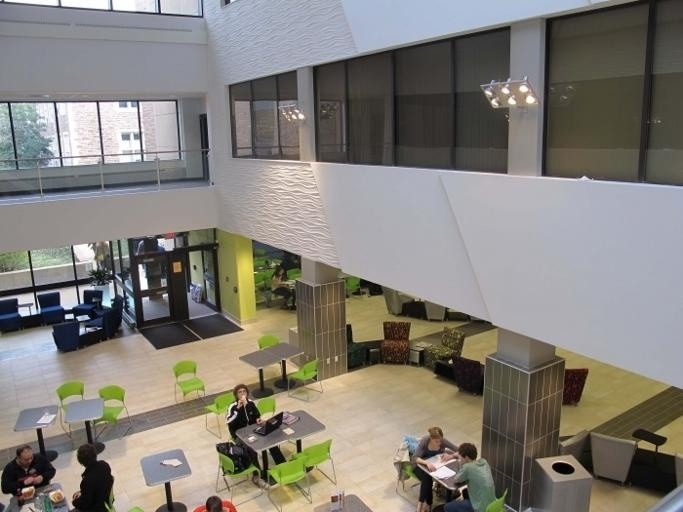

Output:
282,412,299,426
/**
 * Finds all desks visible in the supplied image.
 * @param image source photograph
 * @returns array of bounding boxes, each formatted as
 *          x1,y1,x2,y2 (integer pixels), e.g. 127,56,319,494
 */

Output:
235,410,325,486
240,343,304,398
6,398,191,512
419,454,467,512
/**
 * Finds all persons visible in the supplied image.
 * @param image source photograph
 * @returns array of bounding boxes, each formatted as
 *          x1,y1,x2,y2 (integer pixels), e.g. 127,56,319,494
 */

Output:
443,442,496,512
411,427,459,512
68,444,114,512
225,384,287,479
205,496,222,512
1,444,56,496
95,241,110,274
271,266,297,310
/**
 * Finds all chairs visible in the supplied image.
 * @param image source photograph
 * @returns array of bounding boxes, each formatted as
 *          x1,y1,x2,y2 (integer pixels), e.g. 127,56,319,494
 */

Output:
56,381,84,438
0,290,123,351
486,488,507,512
344,275,486,396
396,438,420,493
266,455,312,512
173,360,205,411
94,385,132,440
287,359,323,401
557,427,681,497
253,248,301,308
205,392,236,439
216,453,263,507
295,440,337,495
258,334,281,378
256,397,276,419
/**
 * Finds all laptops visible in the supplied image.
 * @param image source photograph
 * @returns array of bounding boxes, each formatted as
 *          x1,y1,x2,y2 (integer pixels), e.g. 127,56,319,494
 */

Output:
253,411,285,436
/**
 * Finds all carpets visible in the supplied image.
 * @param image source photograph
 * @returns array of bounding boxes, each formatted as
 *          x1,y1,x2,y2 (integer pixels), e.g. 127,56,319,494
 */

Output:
138,322,201,350
182,313,245,339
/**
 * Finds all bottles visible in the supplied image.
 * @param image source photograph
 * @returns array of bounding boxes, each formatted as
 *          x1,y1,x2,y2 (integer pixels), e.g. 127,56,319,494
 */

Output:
17,489,24,505
45,494,53,511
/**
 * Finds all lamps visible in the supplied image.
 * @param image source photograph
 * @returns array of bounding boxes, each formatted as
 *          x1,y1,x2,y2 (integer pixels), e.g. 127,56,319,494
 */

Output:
278,105,307,123
480,76,541,108
